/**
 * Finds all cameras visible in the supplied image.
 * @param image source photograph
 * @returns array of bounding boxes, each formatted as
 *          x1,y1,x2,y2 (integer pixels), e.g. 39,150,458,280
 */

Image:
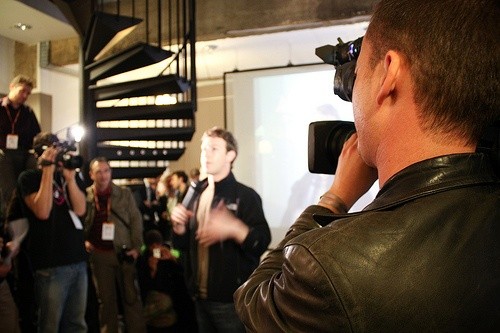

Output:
32,142,83,169
117,247,134,264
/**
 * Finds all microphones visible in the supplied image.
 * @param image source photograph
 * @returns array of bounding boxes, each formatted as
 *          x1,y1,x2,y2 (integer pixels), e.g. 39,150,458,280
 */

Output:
182,180,199,207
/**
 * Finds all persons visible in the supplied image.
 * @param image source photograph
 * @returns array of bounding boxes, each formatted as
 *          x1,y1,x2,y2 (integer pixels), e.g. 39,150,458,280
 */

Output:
170,128,271,333
234,0,500,333
0,75,43,262
0,132,199,333
